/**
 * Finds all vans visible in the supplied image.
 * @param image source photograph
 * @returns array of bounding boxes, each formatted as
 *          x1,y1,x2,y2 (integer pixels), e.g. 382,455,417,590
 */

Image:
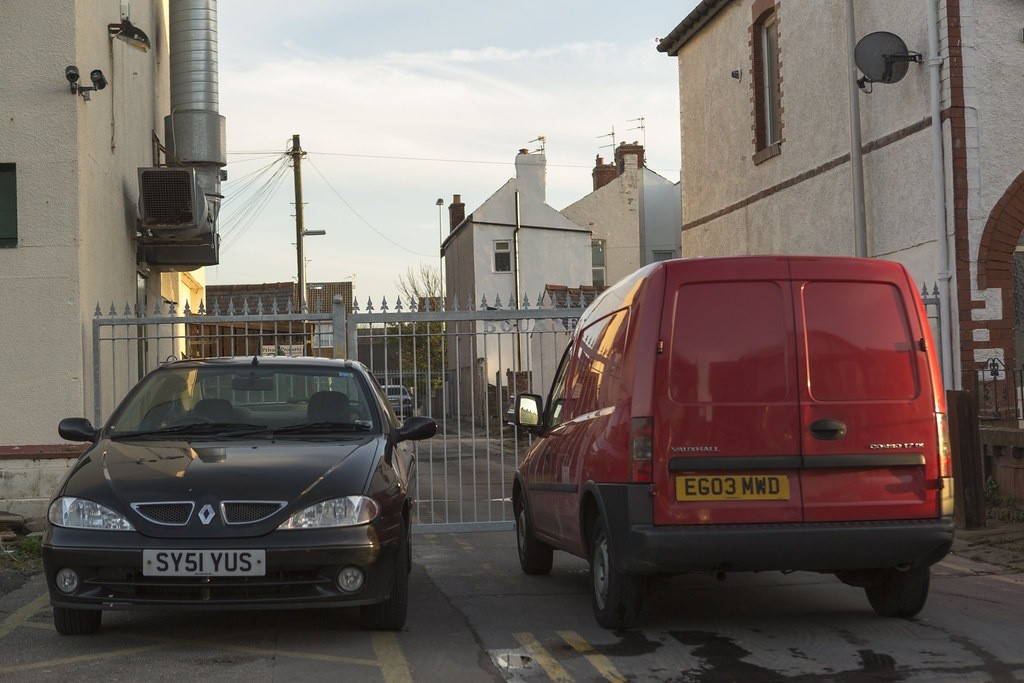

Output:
508,254,958,629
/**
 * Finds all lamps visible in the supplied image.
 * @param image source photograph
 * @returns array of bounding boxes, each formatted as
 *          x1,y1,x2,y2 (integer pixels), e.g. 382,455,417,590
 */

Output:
107,0,151,57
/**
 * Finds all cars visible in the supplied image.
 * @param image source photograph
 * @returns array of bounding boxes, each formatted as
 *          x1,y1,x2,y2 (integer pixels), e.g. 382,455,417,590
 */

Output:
380,384,413,419
39,353,440,637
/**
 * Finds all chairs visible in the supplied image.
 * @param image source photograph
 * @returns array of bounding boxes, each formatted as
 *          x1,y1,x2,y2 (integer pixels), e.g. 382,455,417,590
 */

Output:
305,391,351,428
181,398,236,430
